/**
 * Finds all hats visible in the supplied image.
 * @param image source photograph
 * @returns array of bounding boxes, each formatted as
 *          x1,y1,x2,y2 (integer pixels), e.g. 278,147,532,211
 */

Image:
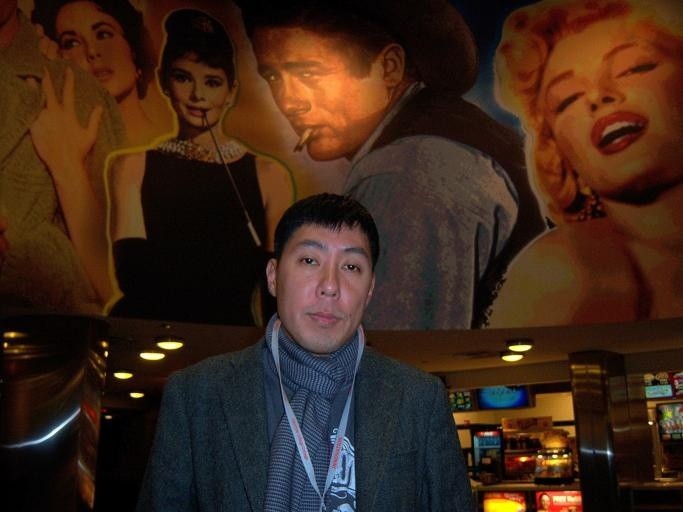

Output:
237,2,479,96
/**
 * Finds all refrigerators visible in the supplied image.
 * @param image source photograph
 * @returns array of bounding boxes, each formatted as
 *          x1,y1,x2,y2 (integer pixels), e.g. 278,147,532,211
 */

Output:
470,425,505,483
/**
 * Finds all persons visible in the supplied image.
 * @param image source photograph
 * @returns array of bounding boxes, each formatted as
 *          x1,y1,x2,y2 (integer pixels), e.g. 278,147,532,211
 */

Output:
238,0,544,331
135,189,475,512
101,1,301,332
22,0,160,311
1,0,126,311
480,0,683,324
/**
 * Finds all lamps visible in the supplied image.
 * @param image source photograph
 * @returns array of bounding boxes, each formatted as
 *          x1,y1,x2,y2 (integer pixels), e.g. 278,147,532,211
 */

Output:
114,369,135,380
500,351,525,362
506,338,535,353
156,336,186,351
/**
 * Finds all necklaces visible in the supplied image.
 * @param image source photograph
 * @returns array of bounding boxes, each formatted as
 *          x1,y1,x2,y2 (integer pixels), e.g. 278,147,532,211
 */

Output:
157,136,246,163
